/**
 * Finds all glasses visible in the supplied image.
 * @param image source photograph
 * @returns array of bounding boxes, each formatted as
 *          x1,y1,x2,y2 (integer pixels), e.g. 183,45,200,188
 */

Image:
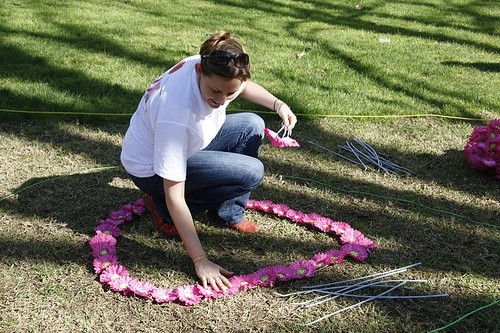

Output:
200,50,251,67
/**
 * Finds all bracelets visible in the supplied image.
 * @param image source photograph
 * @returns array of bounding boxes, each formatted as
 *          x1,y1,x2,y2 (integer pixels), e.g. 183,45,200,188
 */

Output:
276,102,286,114
193,254,206,259
194,257,208,264
273,98,280,111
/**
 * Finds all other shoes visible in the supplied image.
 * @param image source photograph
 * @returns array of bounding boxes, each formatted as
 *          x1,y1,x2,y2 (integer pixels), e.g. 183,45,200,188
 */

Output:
143,193,180,236
221,217,260,234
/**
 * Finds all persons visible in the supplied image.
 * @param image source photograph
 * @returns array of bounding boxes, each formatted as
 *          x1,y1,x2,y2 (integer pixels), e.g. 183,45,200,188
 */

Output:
120,31,297,292
